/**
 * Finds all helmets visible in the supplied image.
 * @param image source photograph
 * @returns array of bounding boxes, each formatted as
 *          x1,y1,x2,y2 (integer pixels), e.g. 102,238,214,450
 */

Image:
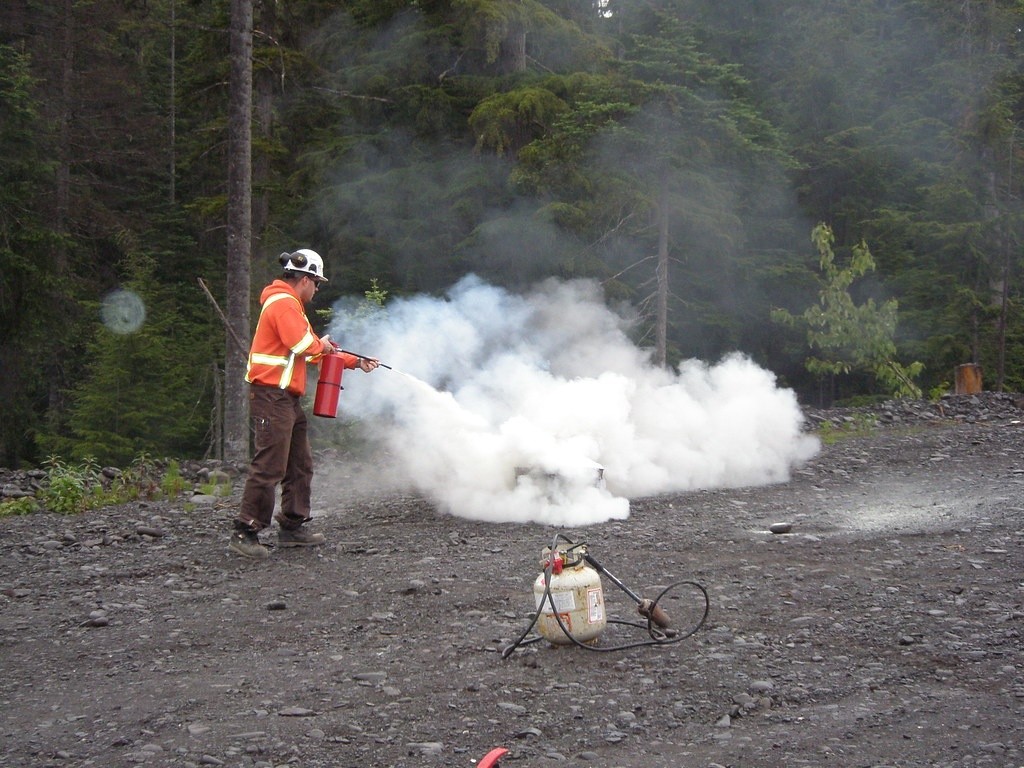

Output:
283,249,328,282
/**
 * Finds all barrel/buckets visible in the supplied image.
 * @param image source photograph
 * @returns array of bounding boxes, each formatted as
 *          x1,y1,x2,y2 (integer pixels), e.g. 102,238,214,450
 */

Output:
955,364,982,394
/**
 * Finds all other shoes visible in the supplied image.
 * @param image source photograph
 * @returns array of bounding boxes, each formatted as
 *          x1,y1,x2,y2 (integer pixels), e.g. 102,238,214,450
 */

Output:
277,526,325,547
229,519,268,559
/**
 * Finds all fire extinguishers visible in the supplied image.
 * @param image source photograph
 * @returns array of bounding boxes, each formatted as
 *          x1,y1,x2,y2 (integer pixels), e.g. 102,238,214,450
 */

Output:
312,340,392,418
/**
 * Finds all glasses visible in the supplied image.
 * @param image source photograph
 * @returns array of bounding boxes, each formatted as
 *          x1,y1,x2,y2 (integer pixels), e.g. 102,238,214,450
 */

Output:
278,252,307,268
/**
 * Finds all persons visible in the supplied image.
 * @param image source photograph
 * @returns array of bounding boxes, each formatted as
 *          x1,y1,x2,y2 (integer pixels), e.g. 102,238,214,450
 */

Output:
227,248,380,558
